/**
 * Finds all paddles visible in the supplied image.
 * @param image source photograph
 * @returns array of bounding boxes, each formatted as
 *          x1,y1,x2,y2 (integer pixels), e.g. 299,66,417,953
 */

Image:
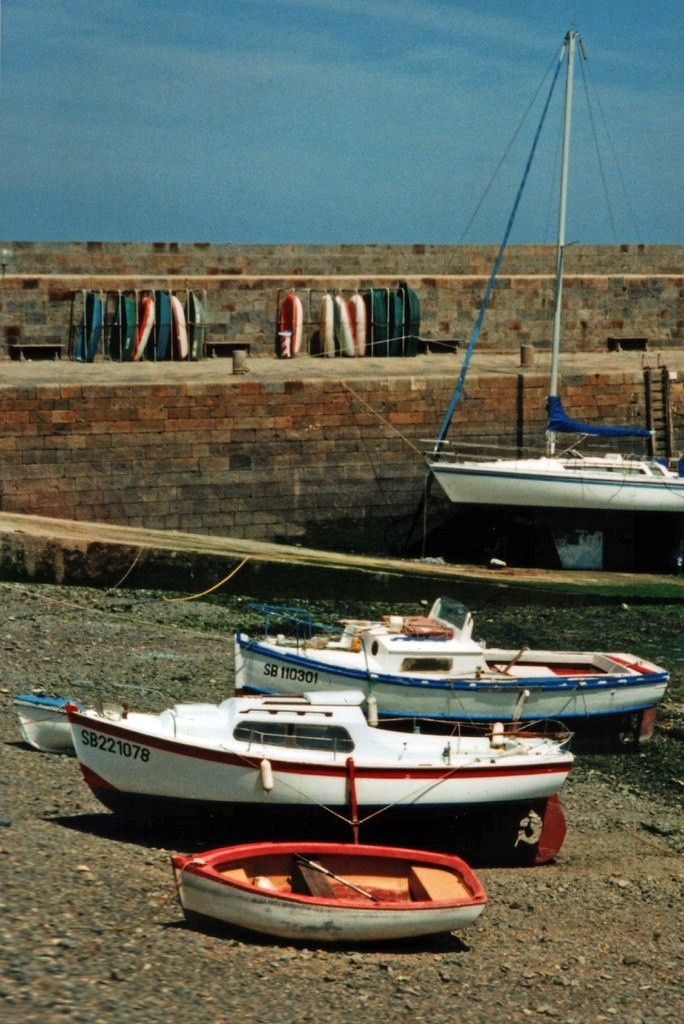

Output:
293,852,384,904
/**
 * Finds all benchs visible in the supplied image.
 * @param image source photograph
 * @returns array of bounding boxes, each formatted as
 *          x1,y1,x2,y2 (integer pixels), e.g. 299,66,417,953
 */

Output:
296,860,338,900
609,335,651,354
206,341,256,359
12,344,66,362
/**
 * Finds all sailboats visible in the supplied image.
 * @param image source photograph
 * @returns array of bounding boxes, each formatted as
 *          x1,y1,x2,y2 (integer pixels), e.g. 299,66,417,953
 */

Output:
419,28,684,526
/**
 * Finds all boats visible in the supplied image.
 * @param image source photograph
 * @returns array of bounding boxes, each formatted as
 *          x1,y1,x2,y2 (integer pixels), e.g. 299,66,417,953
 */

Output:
230,597,669,722
8,691,74,755
66,689,576,864
172,842,486,944
